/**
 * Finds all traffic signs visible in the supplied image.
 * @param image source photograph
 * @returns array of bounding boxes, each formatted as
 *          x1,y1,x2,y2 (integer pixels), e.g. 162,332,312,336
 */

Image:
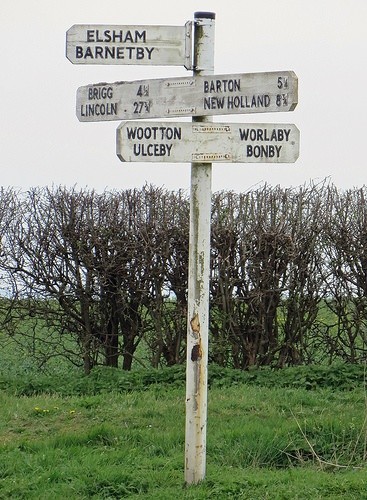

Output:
66,22,302,163
